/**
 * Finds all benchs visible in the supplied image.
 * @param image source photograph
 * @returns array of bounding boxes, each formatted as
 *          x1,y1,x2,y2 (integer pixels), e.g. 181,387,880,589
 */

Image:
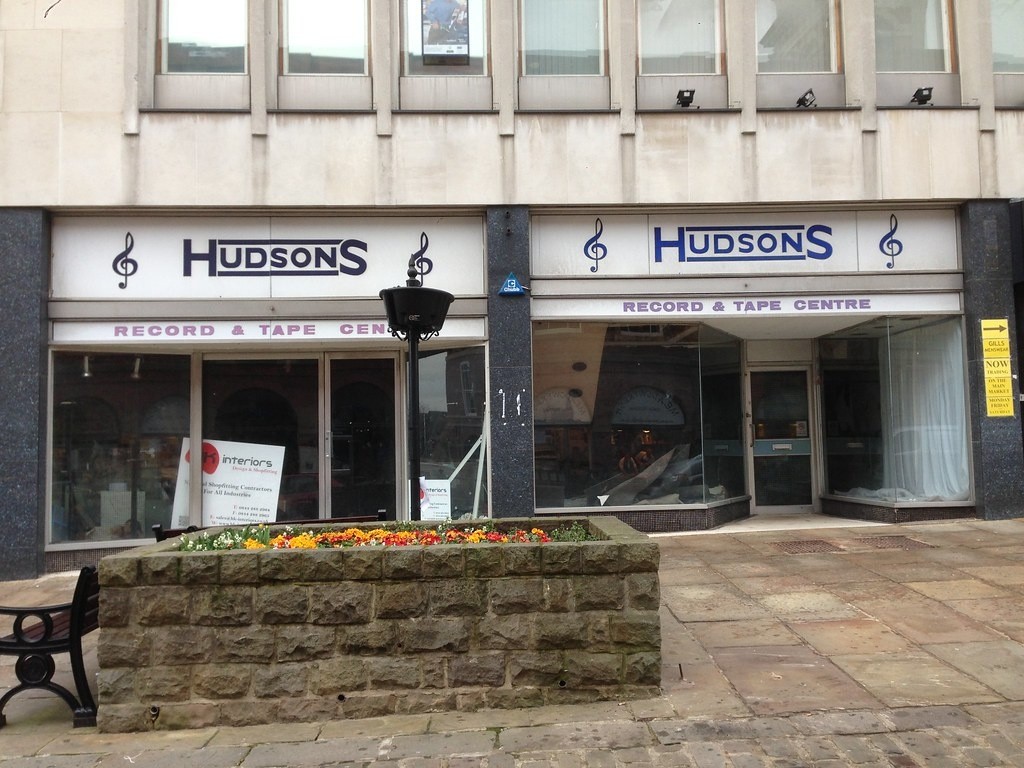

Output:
0,564,98,728
153,509,387,542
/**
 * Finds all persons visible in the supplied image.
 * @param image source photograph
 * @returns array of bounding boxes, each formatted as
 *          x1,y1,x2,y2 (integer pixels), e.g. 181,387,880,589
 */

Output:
425,0,461,45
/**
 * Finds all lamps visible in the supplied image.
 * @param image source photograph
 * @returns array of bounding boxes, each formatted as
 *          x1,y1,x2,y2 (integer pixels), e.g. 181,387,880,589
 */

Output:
128,355,142,379
677,88,695,107
80,355,93,378
909,86,935,107
797,88,818,108
